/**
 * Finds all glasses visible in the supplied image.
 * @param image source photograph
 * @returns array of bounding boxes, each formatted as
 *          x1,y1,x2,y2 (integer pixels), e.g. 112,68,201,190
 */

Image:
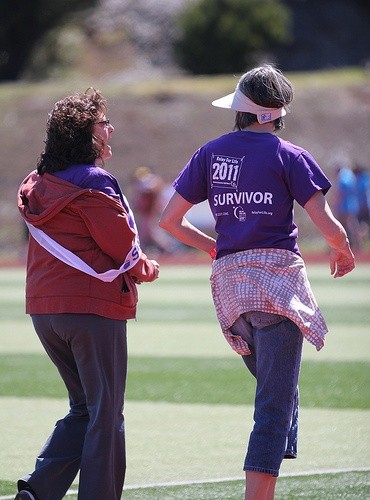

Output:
94,119,111,127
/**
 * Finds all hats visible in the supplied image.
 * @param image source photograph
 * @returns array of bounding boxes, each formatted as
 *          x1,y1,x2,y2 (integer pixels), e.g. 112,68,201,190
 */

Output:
209,82,287,125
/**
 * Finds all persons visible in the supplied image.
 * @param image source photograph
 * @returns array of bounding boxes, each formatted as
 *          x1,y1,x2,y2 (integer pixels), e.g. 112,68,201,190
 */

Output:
14,86,159,500
327,155,369,251
159,61,356,500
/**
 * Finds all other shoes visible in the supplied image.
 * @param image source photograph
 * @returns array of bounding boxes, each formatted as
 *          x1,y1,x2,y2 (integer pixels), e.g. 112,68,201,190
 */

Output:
12,486,35,500
